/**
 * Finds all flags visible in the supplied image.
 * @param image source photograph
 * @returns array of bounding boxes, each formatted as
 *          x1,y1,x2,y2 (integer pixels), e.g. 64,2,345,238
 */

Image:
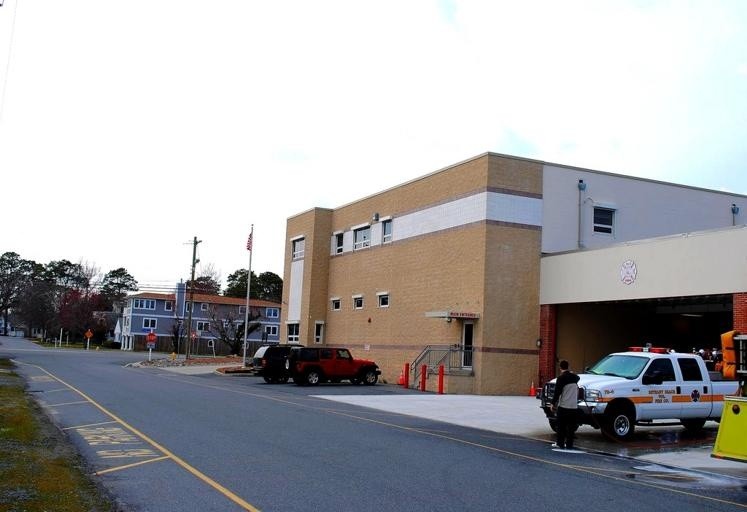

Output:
247,232,252,251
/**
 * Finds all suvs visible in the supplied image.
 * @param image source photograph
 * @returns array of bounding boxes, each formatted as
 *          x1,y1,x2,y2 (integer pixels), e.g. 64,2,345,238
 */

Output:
253,344,307,383
272,356,275,360
286,345,381,387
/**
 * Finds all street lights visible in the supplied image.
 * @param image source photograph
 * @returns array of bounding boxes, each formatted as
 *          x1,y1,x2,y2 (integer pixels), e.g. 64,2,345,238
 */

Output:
185,237,203,357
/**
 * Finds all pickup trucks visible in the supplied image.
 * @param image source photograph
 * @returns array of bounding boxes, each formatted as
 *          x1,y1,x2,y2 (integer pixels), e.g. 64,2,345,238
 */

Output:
539,345,745,443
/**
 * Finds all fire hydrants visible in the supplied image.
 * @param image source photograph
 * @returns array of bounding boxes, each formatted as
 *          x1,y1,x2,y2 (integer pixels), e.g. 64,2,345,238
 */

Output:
171,352,177,361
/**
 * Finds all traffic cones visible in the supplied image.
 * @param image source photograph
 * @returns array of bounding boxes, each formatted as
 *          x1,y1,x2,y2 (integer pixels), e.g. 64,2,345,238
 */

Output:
529,381,536,396
397,370,405,386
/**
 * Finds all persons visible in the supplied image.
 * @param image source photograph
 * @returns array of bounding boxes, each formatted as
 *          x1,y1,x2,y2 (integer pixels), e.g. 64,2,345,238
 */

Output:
552,360,580,450
